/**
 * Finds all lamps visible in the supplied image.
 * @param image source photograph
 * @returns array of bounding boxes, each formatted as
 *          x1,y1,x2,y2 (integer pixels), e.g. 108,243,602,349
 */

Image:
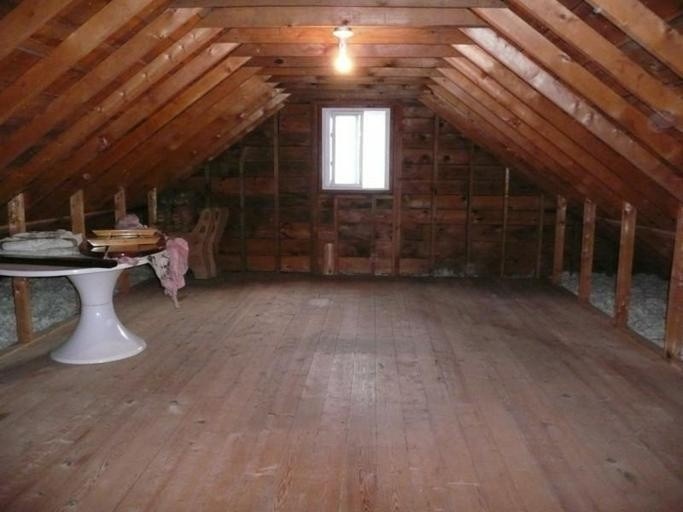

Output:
333,27,354,73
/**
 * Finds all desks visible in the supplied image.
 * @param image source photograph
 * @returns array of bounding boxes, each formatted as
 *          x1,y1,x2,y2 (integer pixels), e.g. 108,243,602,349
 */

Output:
0,248,169,366
